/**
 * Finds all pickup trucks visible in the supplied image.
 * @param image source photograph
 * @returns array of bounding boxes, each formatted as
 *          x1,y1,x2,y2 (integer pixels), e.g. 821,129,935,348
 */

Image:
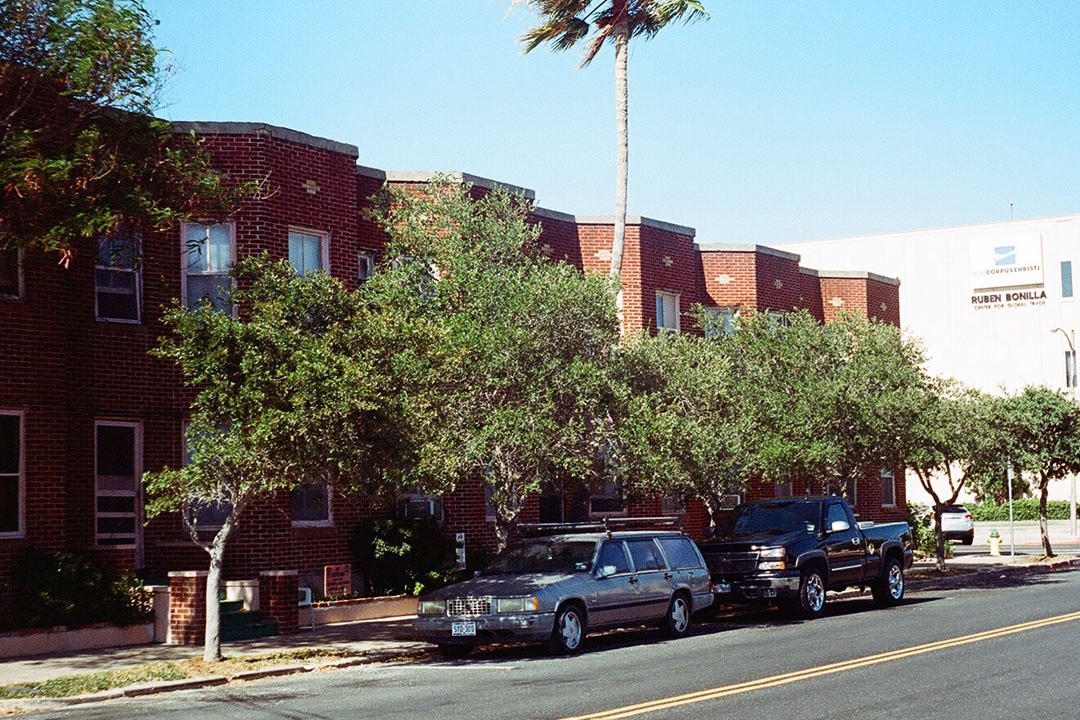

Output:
698,496,915,620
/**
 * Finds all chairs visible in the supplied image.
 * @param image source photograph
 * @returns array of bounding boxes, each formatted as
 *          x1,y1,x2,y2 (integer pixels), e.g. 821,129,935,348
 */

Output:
297,587,316,633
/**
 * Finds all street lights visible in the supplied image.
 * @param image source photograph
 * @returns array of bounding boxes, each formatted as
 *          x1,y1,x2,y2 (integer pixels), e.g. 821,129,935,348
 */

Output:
1049,327,1079,540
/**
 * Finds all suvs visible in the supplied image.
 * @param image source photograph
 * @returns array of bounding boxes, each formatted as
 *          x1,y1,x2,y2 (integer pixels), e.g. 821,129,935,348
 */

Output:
416,515,716,661
924,503,974,544
